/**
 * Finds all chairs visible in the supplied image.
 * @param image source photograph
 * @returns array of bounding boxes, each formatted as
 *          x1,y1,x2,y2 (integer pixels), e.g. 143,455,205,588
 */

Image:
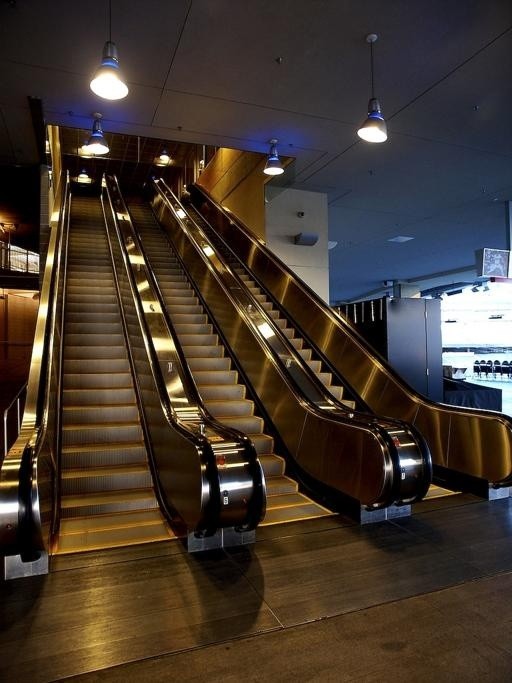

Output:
474,360,512,378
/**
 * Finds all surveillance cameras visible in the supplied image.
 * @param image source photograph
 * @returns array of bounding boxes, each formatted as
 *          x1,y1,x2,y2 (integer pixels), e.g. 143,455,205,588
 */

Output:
298,211,305,218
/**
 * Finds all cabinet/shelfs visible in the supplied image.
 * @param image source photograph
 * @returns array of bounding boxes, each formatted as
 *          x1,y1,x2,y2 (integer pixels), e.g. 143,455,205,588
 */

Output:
329,299,443,405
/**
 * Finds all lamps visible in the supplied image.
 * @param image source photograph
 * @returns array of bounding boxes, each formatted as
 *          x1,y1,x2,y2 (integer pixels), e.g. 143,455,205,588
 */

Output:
86,2,130,103
354,33,389,146
263,139,286,177
87,111,112,157
